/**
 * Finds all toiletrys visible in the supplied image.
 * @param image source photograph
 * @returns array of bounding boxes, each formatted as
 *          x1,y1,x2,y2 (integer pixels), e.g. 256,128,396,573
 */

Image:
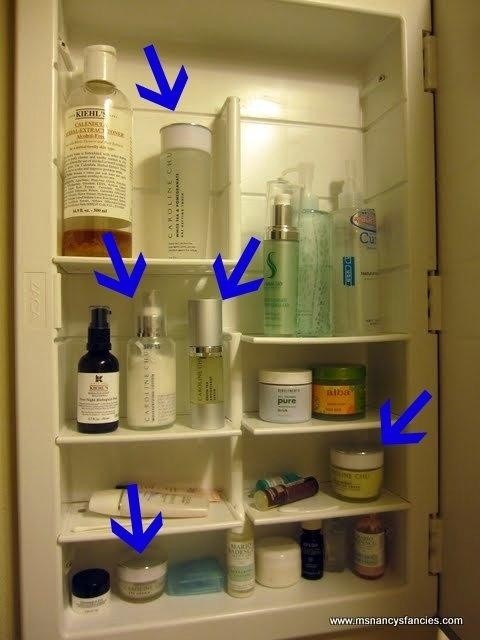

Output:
56,38,396,616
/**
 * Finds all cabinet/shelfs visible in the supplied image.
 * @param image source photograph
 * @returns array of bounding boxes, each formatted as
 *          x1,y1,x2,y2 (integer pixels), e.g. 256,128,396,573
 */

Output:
10,0,453,638
